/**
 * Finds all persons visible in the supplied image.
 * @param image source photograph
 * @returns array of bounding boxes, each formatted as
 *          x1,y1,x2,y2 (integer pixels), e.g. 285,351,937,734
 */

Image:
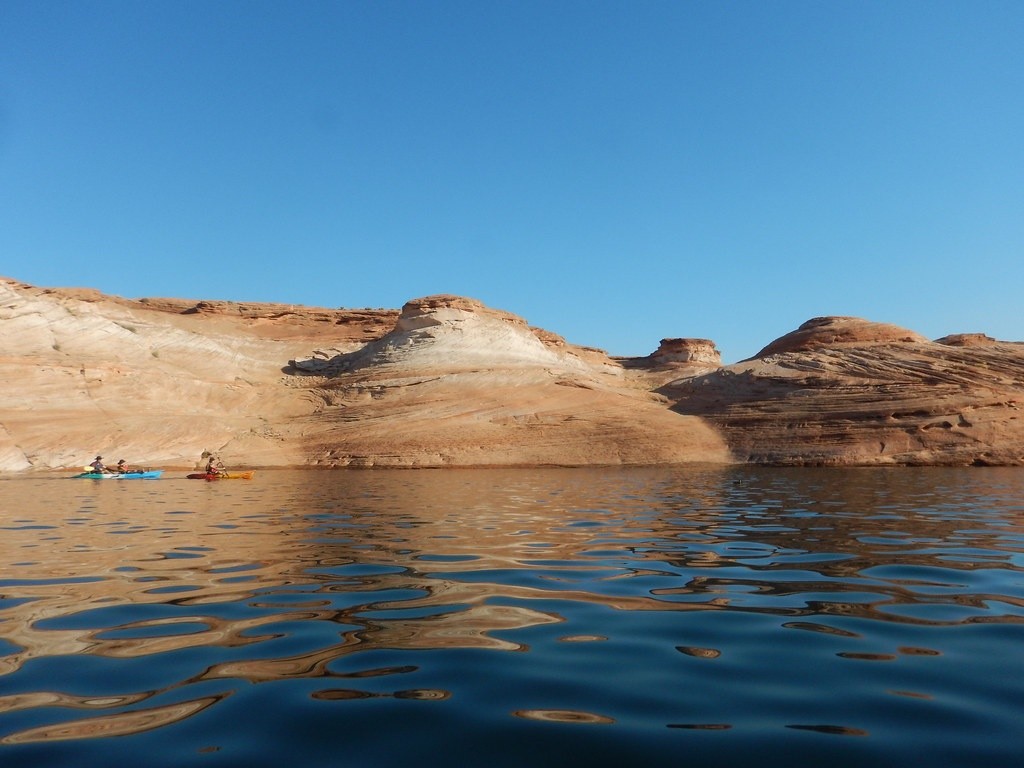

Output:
118,460,128,474
89,456,112,474
205,457,225,474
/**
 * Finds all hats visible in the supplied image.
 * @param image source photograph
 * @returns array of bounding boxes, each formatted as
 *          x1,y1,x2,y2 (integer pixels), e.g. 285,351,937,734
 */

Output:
209,458,215,462
118,459,126,464
95,456,104,459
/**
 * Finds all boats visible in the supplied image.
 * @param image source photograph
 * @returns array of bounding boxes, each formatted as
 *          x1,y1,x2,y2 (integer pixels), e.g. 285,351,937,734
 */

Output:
70,470,164,480
187,470,256,480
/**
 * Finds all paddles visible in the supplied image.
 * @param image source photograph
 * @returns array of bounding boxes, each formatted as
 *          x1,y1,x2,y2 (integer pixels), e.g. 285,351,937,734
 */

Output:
216,454,228,475
84,466,121,473
